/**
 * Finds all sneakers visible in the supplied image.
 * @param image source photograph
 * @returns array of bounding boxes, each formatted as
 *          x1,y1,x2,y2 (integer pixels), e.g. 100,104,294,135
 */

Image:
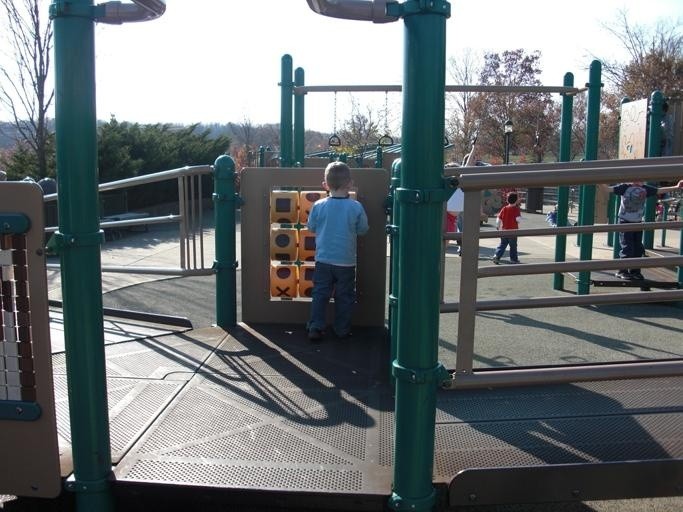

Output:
492,253,521,264
307,329,351,341
614,270,644,280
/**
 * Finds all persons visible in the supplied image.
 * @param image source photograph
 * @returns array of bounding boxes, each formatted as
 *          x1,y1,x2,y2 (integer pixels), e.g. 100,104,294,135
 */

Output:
599,178,683,280
493,191,526,265
445,138,477,246
306,161,370,341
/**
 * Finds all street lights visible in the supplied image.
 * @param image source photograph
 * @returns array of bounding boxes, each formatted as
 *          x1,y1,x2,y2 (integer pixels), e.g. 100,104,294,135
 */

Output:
503,120,514,165
